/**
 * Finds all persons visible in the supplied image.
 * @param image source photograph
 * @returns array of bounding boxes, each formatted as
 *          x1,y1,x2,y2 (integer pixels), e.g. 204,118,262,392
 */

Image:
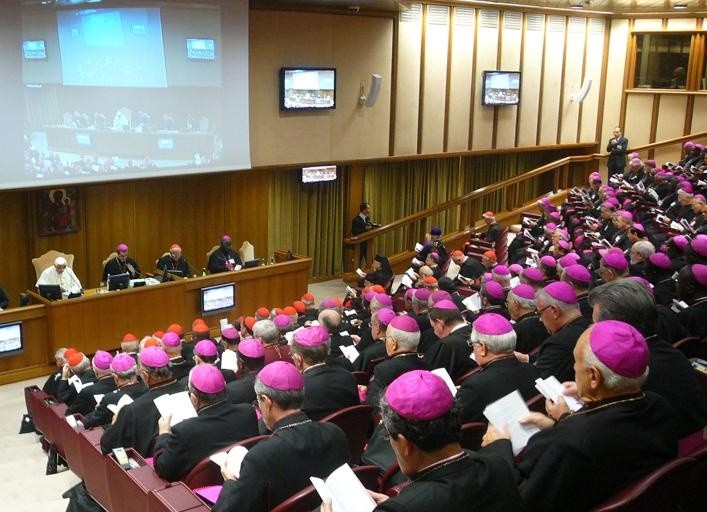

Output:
605,126,627,187
32,140,707,512
18,106,227,179
669,67,686,89
0,287,9,313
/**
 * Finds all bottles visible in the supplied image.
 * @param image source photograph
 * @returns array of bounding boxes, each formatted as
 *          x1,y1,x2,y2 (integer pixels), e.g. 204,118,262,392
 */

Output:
200,267,206,277
260,257,274,266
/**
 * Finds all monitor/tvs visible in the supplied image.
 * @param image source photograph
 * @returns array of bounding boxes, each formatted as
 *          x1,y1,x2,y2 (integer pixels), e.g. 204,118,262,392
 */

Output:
157,137,174,150
0,320,25,357
279,66,337,111
245,259,260,268
108,274,129,291
167,269,184,282
38,284,62,301
482,71,522,105
160,264,168,283
186,37,216,62
201,282,236,318
75,133,90,145
22,39,47,61
286,250,295,261
300,165,337,184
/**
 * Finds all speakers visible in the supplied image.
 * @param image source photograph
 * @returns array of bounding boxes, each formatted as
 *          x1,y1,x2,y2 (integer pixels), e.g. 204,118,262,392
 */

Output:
360,74,382,109
571,78,592,105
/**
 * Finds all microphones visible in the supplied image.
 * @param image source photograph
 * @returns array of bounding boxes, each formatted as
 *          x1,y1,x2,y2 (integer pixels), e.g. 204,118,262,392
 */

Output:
184,257,203,277
126,264,148,279
65,270,86,295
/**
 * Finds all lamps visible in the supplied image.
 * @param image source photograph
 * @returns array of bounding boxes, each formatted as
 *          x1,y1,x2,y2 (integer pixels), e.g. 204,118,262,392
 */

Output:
674,5,688,9
570,4,582,8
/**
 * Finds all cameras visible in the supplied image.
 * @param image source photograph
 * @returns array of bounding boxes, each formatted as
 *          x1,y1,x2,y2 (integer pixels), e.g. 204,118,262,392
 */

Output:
367,221,381,229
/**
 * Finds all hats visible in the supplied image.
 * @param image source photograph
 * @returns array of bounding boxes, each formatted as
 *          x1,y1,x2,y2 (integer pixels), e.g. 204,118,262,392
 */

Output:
475,313,512,335
223,236,231,240
684,142,704,151
431,228,442,236
650,234,707,284
484,240,628,303
255,293,336,315
65,316,182,368
542,197,568,236
118,244,128,251
592,171,645,231
591,320,649,378
363,250,464,332
193,315,304,392
55,258,66,266
385,369,453,420
658,168,703,199
631,153,655,167
486,212,495,216
170,243,179,249
